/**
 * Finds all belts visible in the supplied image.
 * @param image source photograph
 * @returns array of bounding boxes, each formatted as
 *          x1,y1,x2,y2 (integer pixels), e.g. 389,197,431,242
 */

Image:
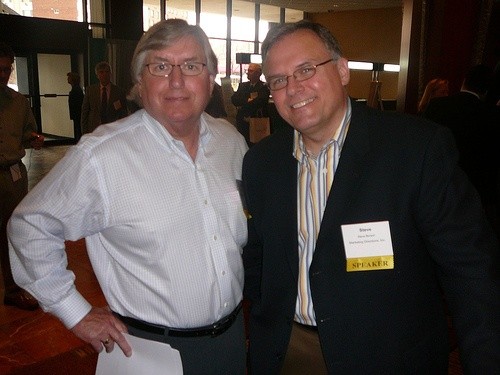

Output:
111,300,244,337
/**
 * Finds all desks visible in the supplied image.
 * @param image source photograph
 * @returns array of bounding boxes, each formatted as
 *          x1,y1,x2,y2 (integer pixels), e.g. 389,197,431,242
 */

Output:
0,238,114,375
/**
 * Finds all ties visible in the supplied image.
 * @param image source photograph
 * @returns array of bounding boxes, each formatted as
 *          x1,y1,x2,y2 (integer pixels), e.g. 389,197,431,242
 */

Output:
101,87,107,111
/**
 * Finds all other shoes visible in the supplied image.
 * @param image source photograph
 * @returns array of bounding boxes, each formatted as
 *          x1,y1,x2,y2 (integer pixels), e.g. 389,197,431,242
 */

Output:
4,289,38,311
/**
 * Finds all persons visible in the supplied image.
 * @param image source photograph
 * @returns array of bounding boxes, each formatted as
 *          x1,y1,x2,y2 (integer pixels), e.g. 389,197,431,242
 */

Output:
417,63,500,241
7,19,250,375
68,61,270,141
0,42,44,310
241,20,500,375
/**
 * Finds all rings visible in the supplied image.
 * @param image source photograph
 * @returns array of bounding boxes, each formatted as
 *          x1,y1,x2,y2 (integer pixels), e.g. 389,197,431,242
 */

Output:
102,338,113,344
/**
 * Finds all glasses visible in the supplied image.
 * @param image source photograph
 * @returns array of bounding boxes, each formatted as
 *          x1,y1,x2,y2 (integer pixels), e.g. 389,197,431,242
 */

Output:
139,62,207,76
264,59,335,90
